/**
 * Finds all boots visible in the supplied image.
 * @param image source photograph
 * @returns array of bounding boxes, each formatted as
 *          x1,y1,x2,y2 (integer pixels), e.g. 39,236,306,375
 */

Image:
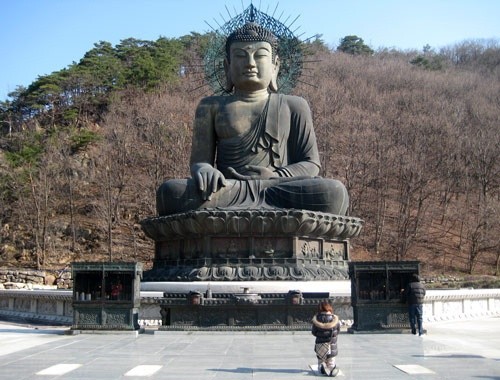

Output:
321,363,330,375
330,364,339,376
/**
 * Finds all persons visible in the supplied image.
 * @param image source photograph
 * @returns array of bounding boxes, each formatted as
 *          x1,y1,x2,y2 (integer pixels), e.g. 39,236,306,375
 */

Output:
312,303,341,376
157,23,348,216
406,275,428,336
